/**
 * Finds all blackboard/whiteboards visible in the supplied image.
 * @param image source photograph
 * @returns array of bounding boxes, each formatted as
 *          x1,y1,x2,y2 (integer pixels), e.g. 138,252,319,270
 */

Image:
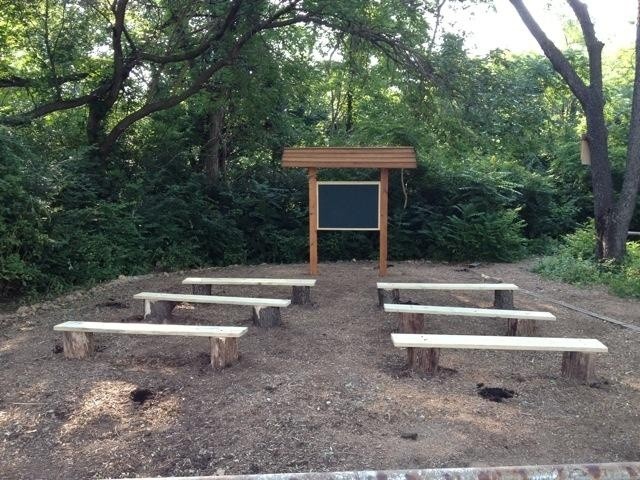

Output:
317,181,381,230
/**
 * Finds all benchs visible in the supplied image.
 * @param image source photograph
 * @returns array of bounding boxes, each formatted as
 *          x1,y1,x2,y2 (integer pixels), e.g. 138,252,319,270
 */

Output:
382,301,556,337
376,283,519,310
183,277,317,305
391,332,607,387
53,320,248,371
134,292,292,329
